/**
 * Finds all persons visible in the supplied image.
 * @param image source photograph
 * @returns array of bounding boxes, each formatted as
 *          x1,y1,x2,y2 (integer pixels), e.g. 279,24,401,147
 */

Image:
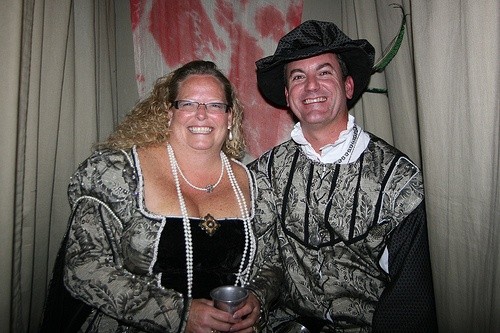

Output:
246,20,439,333
63,60,284,333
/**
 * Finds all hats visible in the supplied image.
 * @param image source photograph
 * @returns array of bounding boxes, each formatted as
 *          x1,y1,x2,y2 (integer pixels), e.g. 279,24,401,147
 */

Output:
255,20,375,110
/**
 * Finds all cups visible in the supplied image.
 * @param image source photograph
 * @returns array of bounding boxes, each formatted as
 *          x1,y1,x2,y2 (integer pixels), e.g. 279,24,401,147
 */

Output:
210,286,248,325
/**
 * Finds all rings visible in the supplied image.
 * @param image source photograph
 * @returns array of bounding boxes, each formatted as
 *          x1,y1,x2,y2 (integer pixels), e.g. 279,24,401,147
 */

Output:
212,329,217,333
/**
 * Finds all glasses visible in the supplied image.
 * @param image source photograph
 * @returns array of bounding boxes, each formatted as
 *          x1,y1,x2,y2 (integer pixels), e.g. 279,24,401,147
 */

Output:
171,100,229,114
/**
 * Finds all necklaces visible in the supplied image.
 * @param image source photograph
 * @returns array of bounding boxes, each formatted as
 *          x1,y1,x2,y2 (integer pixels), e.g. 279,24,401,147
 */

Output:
165,140,257,298
297,124,359,167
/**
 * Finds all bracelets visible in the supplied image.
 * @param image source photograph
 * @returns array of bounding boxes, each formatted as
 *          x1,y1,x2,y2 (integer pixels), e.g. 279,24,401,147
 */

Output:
251,290,264,322
